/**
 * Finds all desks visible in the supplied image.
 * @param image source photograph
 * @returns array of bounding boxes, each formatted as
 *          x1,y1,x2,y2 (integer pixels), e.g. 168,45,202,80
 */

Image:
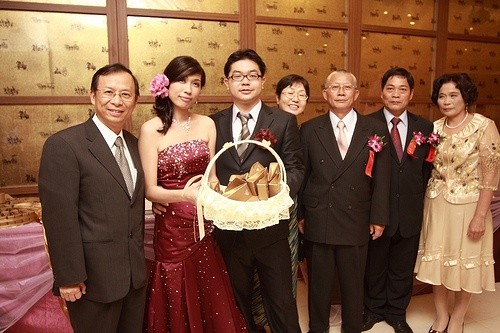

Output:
0,197,156,332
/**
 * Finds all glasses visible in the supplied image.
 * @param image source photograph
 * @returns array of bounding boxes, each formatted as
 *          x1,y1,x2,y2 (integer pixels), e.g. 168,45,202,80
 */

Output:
96,86,135,102
325,82,357,92
228,73,262,81
282,90,308,101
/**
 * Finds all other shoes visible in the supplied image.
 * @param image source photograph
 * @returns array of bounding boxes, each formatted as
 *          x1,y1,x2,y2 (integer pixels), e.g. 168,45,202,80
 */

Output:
429,312,465,333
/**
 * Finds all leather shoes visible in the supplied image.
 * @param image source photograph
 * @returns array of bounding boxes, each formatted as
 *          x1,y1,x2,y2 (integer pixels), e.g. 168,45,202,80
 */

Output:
362,316,385,331
385,319,413,333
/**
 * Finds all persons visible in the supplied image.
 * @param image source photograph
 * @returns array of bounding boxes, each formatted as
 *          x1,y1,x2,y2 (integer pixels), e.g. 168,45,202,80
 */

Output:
38,64,149,333
251,74,309,333
413,73,500,333
137,55,248,333
363,67,434,333
152,50,306,333
297,71,391,333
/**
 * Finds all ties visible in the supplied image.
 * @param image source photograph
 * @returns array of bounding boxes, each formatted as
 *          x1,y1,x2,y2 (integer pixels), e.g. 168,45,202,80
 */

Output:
390,117,404,166
336,121,349,160
114,137,134,200
237,112,253,163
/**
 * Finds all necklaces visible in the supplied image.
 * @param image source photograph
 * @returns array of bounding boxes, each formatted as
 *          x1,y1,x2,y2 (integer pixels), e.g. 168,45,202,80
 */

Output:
445,110,468,129
171,113,192,136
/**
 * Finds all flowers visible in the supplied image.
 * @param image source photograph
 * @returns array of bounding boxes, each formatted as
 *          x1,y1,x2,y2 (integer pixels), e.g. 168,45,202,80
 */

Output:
149,73,169,98
367,133,388,152
427,131,441,147
252,128,276,149
413,131,426,146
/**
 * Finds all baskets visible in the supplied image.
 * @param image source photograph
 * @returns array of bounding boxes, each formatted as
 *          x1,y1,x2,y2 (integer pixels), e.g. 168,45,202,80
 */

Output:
197,140,294,230
